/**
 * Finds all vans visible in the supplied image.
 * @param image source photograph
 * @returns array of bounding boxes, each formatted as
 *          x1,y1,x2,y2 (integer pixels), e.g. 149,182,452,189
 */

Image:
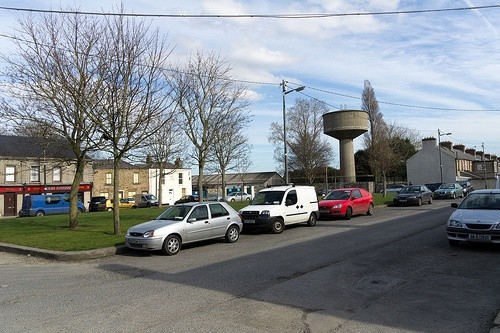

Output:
133,194,159,207
238,185,320,234
19,194,86,217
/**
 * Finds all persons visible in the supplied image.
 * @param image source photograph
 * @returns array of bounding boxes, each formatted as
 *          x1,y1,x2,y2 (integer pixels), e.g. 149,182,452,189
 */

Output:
409,182,413,186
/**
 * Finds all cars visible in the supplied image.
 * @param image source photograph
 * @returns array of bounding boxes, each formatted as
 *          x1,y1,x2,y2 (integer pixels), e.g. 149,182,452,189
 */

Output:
446,189,500,248
125,201,243,256
98,199,138,211
425,181,474,200
174,194,200,205
226,192,252,202
203,194,222,202
380,184,433,207
319,188,375,220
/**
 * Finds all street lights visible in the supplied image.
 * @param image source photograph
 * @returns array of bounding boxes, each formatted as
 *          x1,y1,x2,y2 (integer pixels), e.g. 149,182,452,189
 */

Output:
282,80,305,186
438,129,452,183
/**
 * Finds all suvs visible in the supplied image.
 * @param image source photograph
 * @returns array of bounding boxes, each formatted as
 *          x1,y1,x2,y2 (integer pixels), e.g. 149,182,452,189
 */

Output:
87,196,107,211
